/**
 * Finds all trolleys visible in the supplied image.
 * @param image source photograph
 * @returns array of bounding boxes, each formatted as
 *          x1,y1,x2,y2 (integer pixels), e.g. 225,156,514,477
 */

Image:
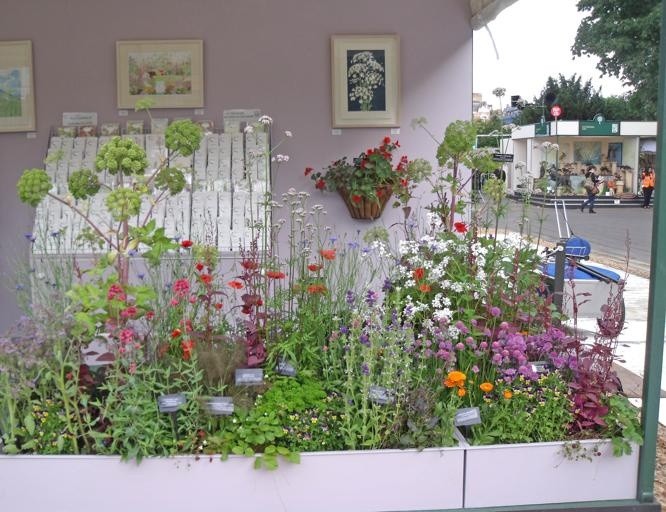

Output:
529,201,625,337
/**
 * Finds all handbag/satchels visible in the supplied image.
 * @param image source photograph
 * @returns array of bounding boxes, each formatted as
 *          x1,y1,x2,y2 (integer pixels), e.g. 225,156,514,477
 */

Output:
584,173,595,191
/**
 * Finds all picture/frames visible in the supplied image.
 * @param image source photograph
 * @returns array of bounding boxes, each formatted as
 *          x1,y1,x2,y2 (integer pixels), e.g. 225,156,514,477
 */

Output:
328,34,403,131
1,38,40,136
112,38,205,112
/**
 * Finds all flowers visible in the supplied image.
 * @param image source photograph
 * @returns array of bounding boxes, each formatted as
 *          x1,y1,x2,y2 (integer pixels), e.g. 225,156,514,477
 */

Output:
303,137,417,207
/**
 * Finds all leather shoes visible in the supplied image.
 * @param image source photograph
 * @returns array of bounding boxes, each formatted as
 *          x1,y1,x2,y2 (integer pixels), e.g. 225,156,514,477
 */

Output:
589,210,596,213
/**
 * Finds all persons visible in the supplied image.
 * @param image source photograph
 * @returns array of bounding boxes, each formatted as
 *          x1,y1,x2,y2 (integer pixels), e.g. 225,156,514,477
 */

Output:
579,165,604,214
638,165,656,209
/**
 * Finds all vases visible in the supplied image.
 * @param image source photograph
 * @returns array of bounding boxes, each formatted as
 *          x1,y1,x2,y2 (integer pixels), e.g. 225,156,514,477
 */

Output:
336,185,394,220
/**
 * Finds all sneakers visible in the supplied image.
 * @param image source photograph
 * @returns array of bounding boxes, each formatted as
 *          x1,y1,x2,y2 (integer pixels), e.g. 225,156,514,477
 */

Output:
643,205,650,208
